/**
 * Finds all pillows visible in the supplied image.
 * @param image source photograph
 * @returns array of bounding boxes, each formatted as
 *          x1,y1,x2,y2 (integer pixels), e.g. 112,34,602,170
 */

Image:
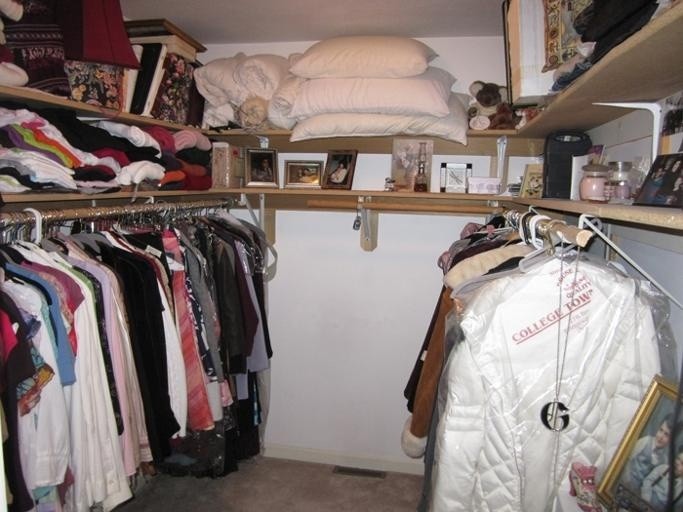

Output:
285,32,473,146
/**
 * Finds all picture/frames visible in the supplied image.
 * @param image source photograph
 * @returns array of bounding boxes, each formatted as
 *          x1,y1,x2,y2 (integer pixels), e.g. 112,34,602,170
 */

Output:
244,147,358,189
594,374,682,512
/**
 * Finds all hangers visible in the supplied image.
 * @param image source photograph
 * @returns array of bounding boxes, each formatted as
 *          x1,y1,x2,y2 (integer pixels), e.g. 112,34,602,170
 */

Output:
0,199,268,335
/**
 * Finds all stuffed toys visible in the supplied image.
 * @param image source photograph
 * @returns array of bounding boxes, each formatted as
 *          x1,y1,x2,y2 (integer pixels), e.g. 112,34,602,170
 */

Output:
467,80,508,130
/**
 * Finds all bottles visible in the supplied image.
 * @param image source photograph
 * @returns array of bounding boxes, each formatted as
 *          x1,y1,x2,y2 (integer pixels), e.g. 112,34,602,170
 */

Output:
414,160,428,193
607,161,634,204
603,180,630,205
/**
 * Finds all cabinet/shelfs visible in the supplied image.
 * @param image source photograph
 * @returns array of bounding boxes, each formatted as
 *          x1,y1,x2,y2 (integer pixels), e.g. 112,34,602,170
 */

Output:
1,84,206,213
208,114,515,224
514,2,683,232
436,204,662,336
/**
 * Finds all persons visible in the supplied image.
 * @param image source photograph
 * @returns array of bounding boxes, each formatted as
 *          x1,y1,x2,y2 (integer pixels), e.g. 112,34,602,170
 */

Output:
636,158,683,208
327,158,349,185
561,1,578,46
621,413,683,512
262,158,272,181
296,167,313,181
